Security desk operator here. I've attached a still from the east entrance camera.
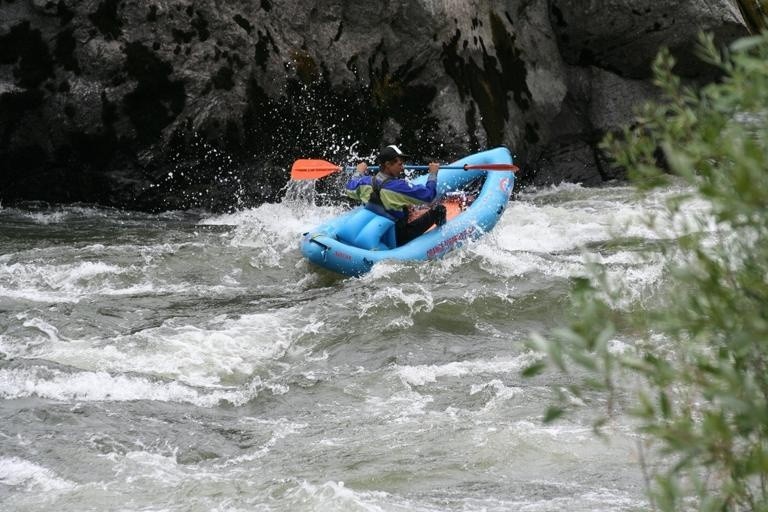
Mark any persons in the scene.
[343,145,446,247]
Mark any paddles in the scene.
[290,159,519,180]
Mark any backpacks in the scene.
[369,176,393,208]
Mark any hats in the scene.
[381,145,410,161]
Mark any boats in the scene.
[301,147,516,276]
